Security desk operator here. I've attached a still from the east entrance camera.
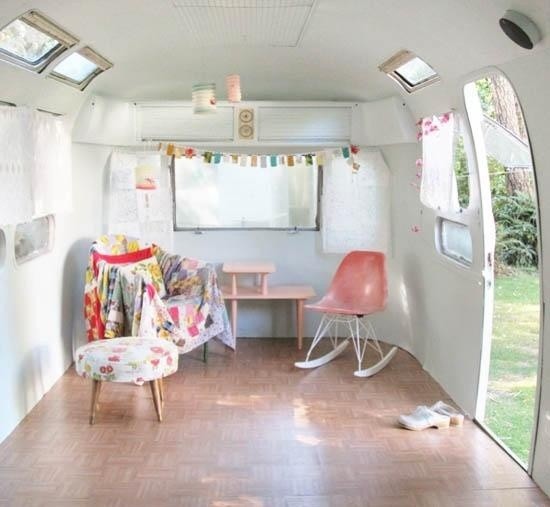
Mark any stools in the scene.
[76,336,178,424]
[222,260,276,295]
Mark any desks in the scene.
[219,285,316,350]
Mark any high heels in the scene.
[398,406,451,431]
[429,400,464,425]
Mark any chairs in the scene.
[294,251,398,378]
[84,233,218,362]
